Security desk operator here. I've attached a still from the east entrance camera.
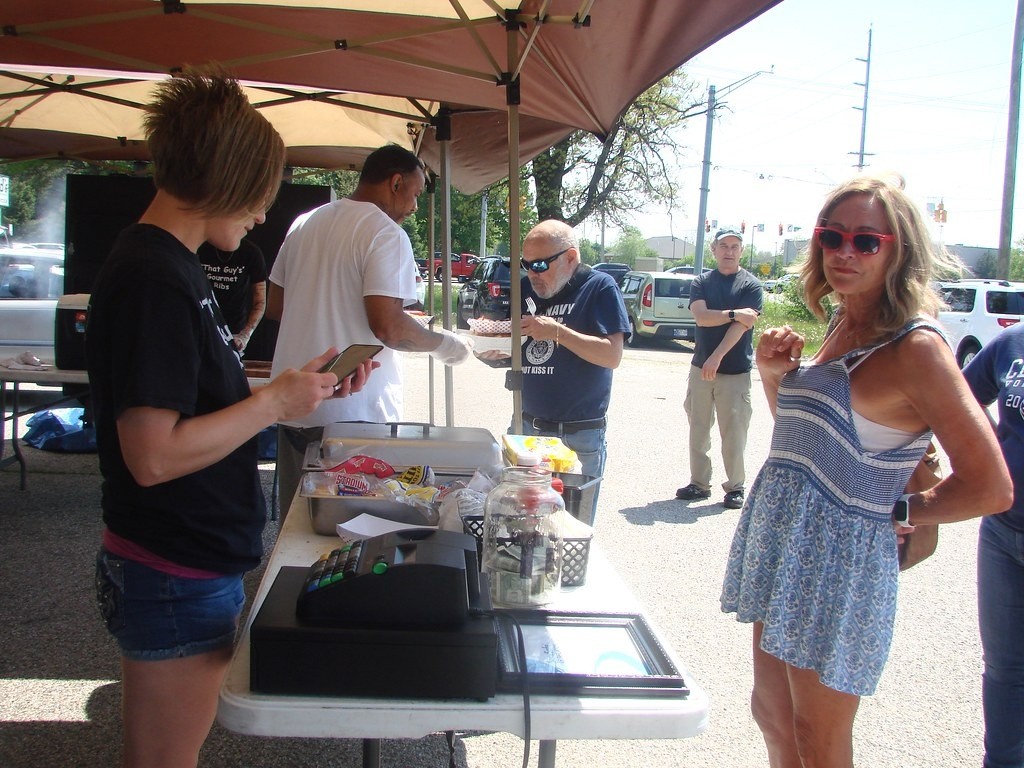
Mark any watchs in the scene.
[729,309,735,323]
[894,494,916,528]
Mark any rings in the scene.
[790,356,801,362]
[349,392,354,398]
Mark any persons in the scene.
[196,238,268,352]
[472,220,632,528]
[720,180,1014,768]
[83,61,380,768]
[961,322,1024,768]
[267,145,475,541]
[675,225,764,509]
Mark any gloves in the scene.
[428,329,474,367]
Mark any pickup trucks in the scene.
[426,253,481,283]
[415,251,447,278]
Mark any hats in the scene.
[715,226,743,241]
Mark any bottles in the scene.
[481,465,562,607]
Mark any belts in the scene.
[522,412,608,434]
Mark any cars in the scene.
[928,277,1024,372]
[1,242,89,390]
[664,266,713,276]
[619,271,701,346]
[592,263,632,285]
[765,274,799,293]
[455,257,531,329]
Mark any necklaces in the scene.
[844,317,876,340]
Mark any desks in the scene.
[0,363,278,521]
[217,473,705,768]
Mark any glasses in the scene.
[520,247,575,273]
[815,227,896,256]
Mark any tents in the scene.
[0,0,779,436]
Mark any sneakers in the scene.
[675,483,712,500]
[724,491,744,508]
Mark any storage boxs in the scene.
[55,293,90,370]
[461,515,593,586]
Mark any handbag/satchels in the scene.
[899,440,944,573]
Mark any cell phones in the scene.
[318,344,384,387]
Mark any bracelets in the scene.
[557,322,559,348]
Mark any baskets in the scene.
[461,515,595,586]
